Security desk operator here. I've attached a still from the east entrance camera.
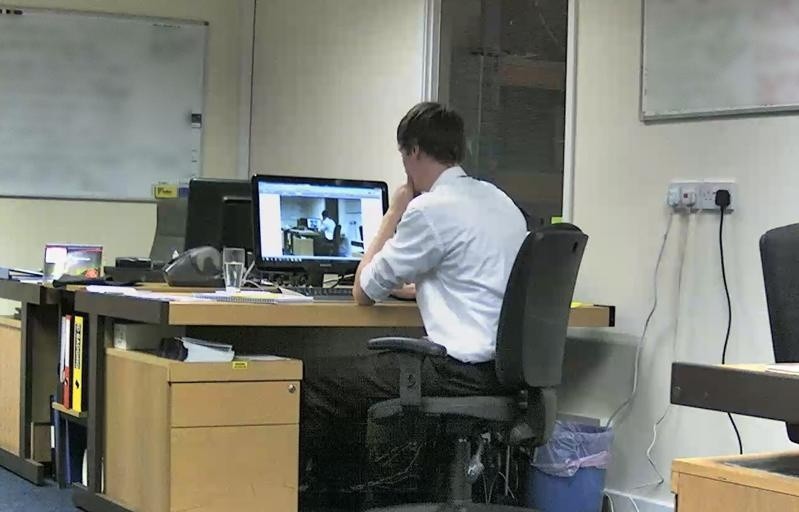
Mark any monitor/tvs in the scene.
[182,176,254,252]
[306,217,322,231]
[297,218,306,226]
[250,173,390,288]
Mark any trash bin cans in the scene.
[529,422,614,512]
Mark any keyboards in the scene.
[286,285,354,301]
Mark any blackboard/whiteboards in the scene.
[0,5,210,204]
[638,1,799,122]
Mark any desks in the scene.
[66,285,616,512]
[1,267,162,491]
[661,356,797,434]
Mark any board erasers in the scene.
[152,184,179,198]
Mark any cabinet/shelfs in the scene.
[670,451,798,511]
[2,316,54,462]
[103,345,304,512]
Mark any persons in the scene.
[302,102,527,509]
[317,210,337,250]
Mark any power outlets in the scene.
[701,180,735,211]
[667,182,699,211]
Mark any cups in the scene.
[222,246,255,294]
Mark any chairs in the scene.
[752,221,797,451]
[144,196,189,273]
[362,223,589,510]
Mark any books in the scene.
[58,317,65,404]
[71,314,86,411]
[6,267,43,277]
[63,314,74,410]
[193,292,313,305]
[159,335,236,362]
